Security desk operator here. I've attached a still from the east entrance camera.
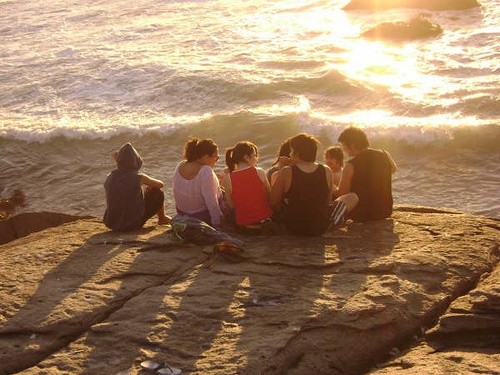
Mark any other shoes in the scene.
[158,216,171,224]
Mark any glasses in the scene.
[214,154,219,161]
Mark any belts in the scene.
[246,224,262,229]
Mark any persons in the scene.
[173,137,236,231]
[265,140,291,184]
[103,141,174,233]
[323,145,345,192]
[225,140,281,234]
[330,125,397,222]
[271,133,334,237]
